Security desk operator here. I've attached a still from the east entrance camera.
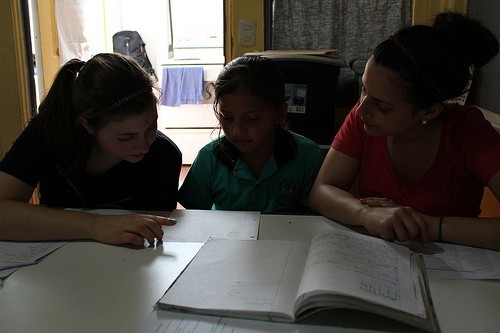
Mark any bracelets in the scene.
[438,216,444,243]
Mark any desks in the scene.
[0,210,500,333]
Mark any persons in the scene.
[309,9,500,253]
[0,54,182,247]
[178,57,324,215]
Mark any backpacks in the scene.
[113,30,152,74]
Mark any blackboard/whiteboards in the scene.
[169,0,224,49]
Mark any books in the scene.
[154,228,441,333]
